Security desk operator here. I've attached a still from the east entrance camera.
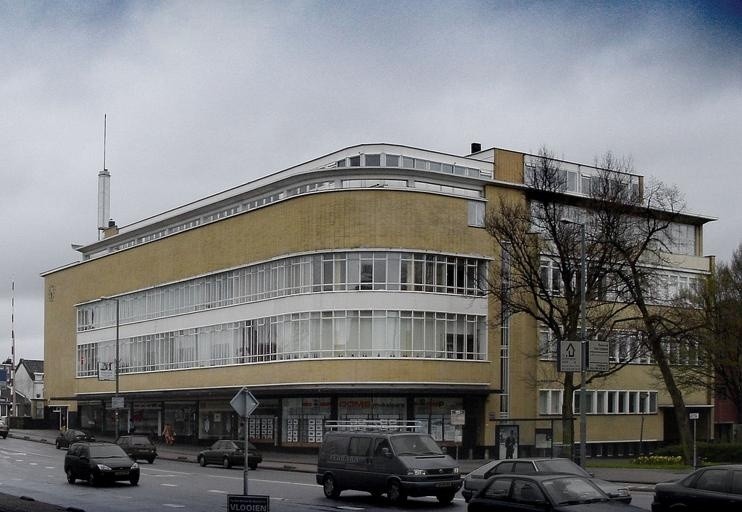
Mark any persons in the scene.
[238,423,244,440]
[60,424,69,437]
[161,423,172,445]
[504,429,516,459]
[130,417,135,435]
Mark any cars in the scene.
[462,457,650,511]
[651,465,742,512]
[115,435,158,464]
[196,439,263,470]
[0,418,8,439]
[55,428,96,450]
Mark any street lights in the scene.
[99,296,121,443]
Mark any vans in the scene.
[316,431,464,505]
[64,439,139,487]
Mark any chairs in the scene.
[520,481,567,500]
